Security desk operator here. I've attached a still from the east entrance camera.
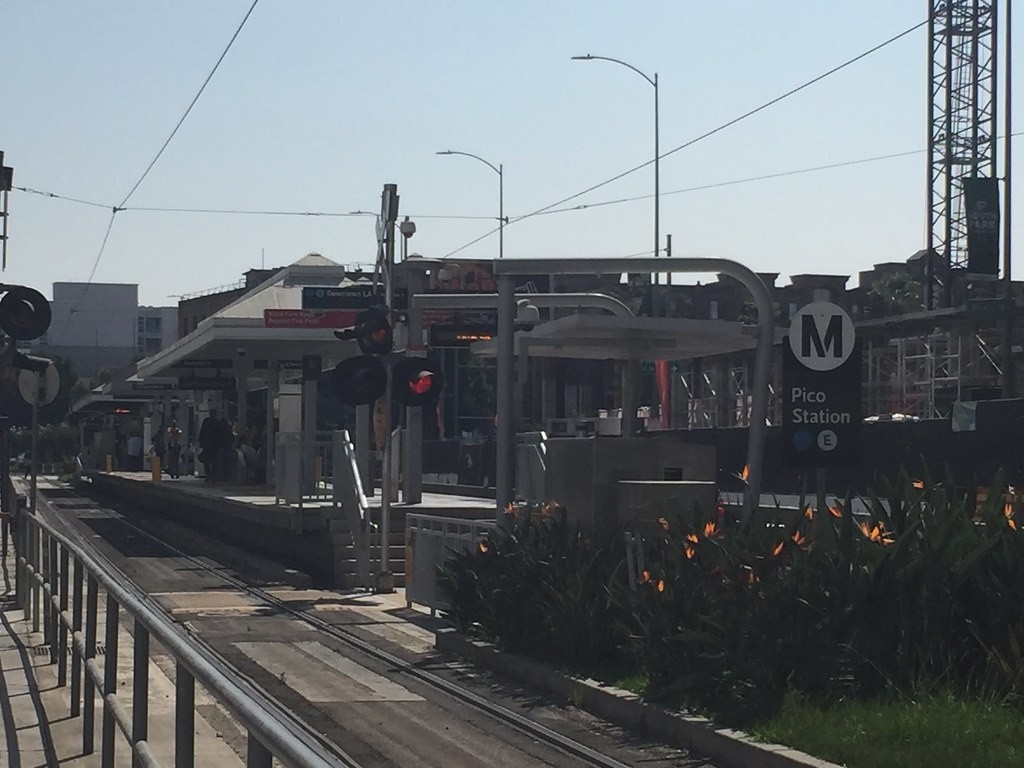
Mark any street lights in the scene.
[571,51,660,285]
[436,153,511,261]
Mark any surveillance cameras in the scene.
[517,306,540,332]
[400,222,416,238]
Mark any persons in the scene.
[127,418,183,479]
[198,407,234,485]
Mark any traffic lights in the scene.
[388,354,444,411]
[334,301,393,358]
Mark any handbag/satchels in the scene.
[198,447,208,462]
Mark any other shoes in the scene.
[204,474,211,483]
